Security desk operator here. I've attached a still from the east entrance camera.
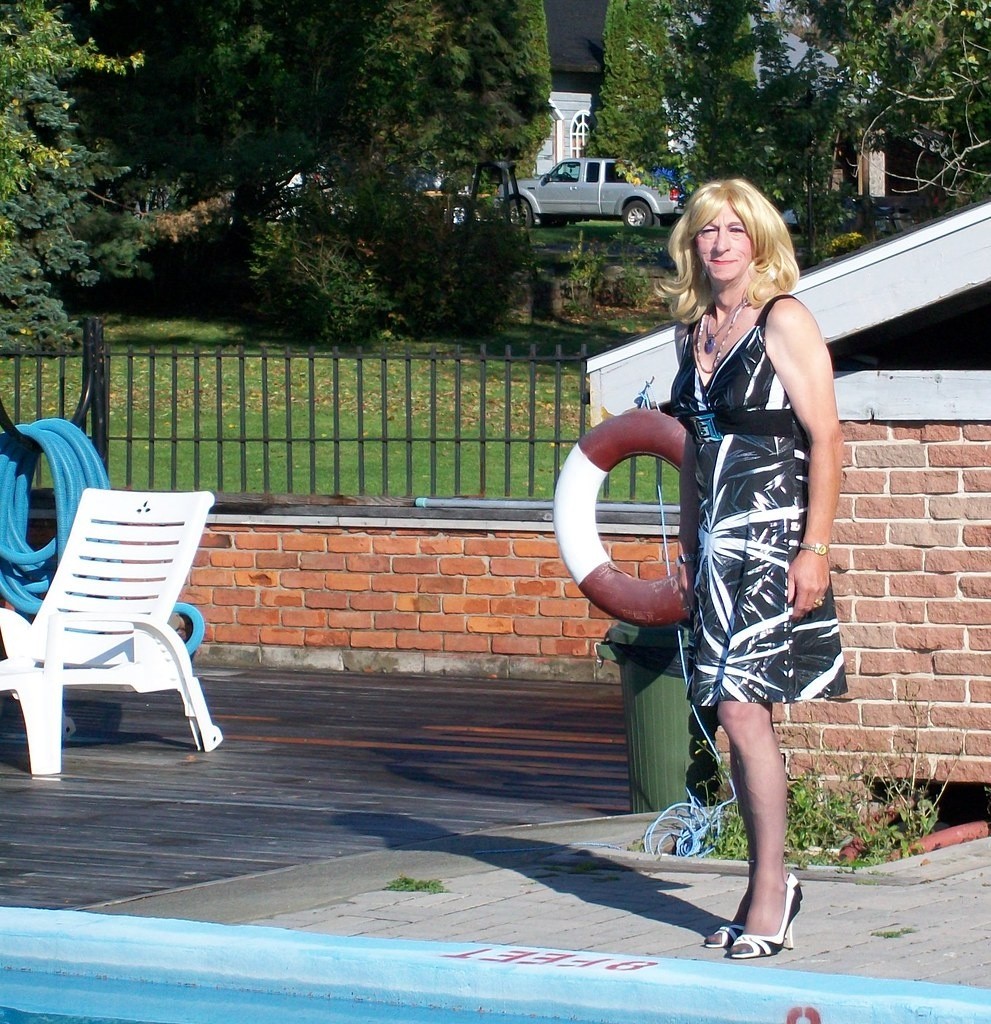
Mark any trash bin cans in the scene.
[596,621,719,814]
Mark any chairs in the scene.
[0,484,226,779]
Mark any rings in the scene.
[814,599,823,606]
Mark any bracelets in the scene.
[675,553,697,568]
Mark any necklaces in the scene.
[705,300,746,354]
[696,301,747,373]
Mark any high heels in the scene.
[731,882,800,959]
[705,873,803,949]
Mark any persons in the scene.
[654,180,849,958]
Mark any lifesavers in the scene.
[552,411,693,626]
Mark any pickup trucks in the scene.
[498,158,691,230]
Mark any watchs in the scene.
[800,542,828,555]
